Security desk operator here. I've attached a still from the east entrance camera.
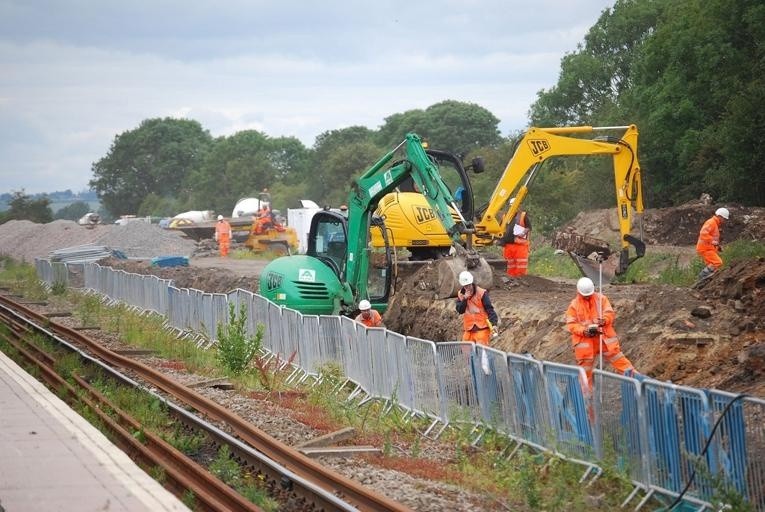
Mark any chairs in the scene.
[327,241,346,260]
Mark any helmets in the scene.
[358,299,372,311]
[714,207,730,220]
[458,271,474,286]
[217,215,224,221]
[576,277,594,297]
[509,198,516,205]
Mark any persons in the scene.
[694,207,730,278]
[493,197,533,276]
[353,299,382,336]
[251,204,287,235]
[455,271,499,371]
[215,215,231,257]
[566,277,640,426]
